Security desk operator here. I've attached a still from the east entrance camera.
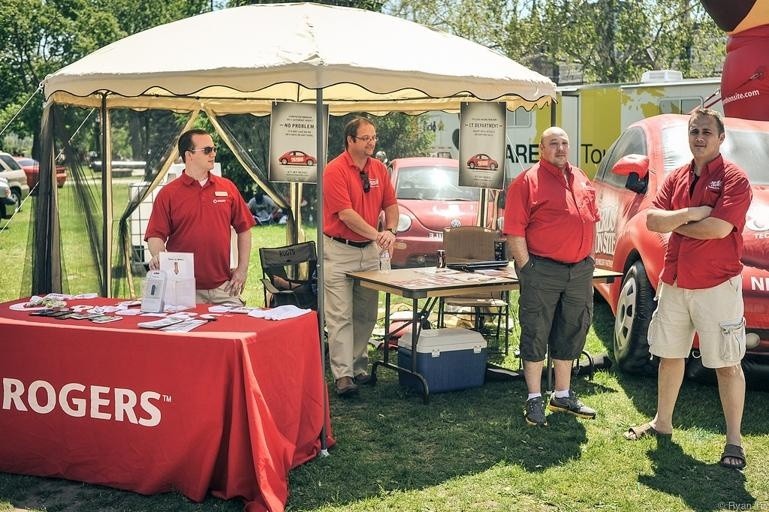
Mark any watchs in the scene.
[382,226,396,235]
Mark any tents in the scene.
[39,0,559,393]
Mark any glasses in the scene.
[360,171,370,193]
[188,147,217,155]
[350,135,379,142]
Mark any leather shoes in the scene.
[353,373,378,385]
[335,376,360,396]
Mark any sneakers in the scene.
[548,389,596,418]
[523,396,548,426]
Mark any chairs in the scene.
[438,226,510,355]
[259,242,330,348]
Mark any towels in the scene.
[248,304,312,321]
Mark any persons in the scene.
[145,129,258,310]
[316,119,401,396]
[619,107,754,469]
[503,126,601,427]
[246,186,276,226]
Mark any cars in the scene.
[378,157,504,268]
[467,152,499,171]
[593,111,768,378]
[13,156,67,192]
[279,150,318,167]
[0,152,30,214]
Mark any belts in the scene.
[529,253,588,265]
[323,232,373,248]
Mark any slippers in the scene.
[622,423,672,440]
[720,444,746,468]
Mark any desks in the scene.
[0,292,318,499]
[342,266,624,405]
